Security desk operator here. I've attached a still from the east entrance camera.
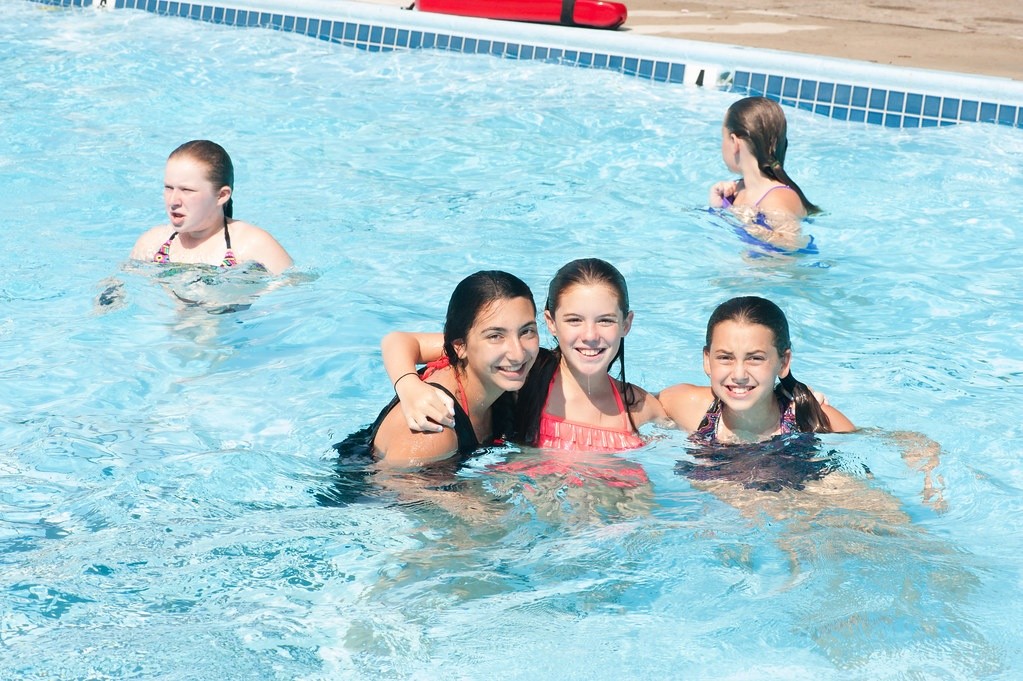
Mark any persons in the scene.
[709,96,822,216]
[381,258,672,459]
[333,270,539,469]
[129,140,292,276]
[653,296,856,443]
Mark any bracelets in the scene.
[393,371,423,390]
